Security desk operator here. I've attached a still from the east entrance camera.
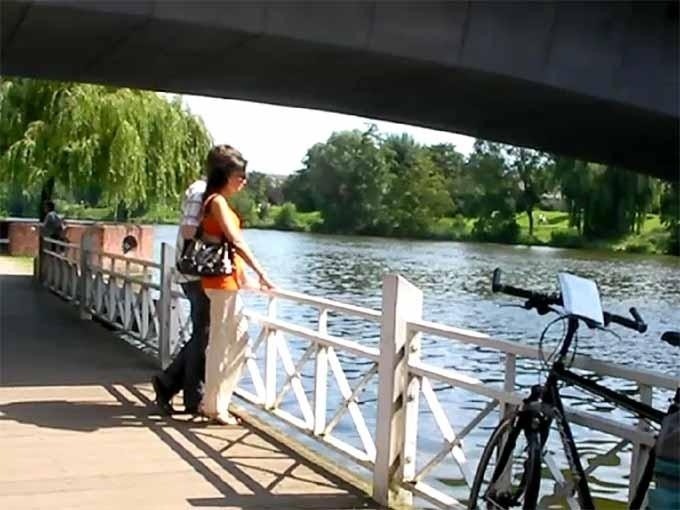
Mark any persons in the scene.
[197,147,276,427]
[151,143,242,418]
[32,200,66,258]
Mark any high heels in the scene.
[197,402,242,424]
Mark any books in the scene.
[557,269,607,327]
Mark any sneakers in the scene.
[185,403,200,414]
[152,376,176,415]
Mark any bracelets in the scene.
[258,271,265,279]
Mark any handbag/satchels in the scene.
[176,238,234,276]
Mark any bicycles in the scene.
[466,266,679,509]
